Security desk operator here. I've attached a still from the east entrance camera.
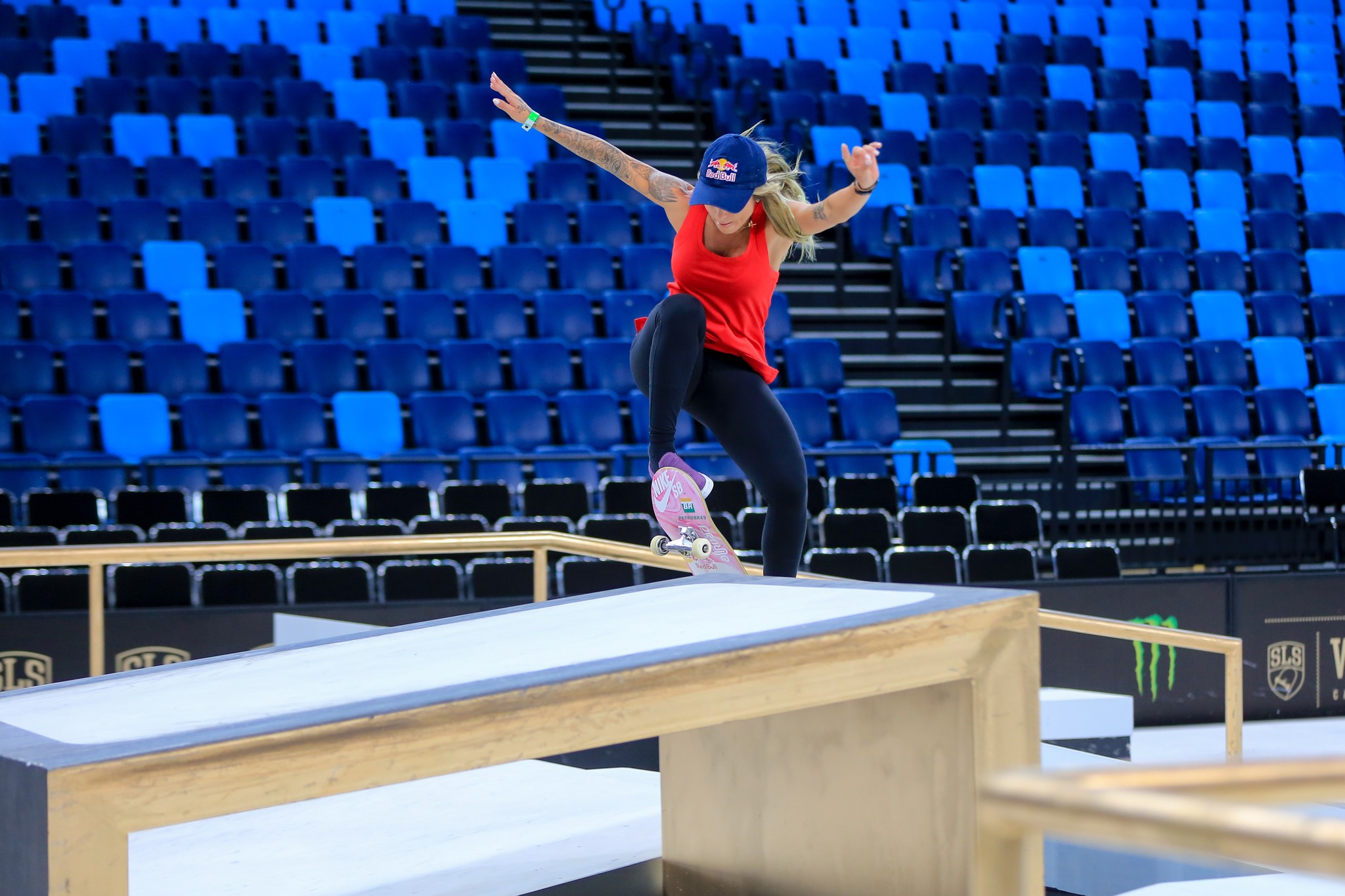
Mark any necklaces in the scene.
[737,220,757,230]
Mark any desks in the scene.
[0,571,1047,895]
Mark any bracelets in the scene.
[853,181,878,195]
[521,110,540,132]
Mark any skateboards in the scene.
[650,466,748,576]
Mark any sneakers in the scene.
[648,452,713,499]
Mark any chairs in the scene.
[0,1,1345,618]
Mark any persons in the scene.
[490,71,882,577]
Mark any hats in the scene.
[689,134,767,214]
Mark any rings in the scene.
[844,156,850,162]
